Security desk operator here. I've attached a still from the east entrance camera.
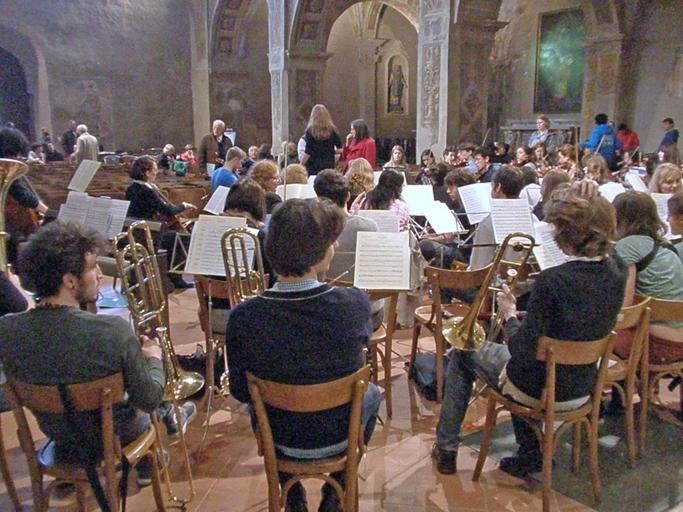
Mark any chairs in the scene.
[244,363,372,512]
[408,262,496,403]
[19,148,212,218]
[571,295,654,470]
[321,276,402,417]
[500,259,540,280]
[0,372,165,511]
[471,330,618,512]
[193,273,270,353]
[633,290,683,458]
[0,433,23,511]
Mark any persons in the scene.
[282,163,307,185]
[279,144,299,168]
[457,143,479,175]
[473,146,497,182]
[582,151,611,186]
[313,170,385,333]
[248,160,282,215]
[641,153,659,187]
[417,150,439,185]
[528,116,558,155]
[515,146,536,170]
[0,223,196,485]
[180,145,202,173]
[581,114,620,173]
[1,128,48,263]
[257,144,273,160]
[342,158,374,211]
[383,145,416,184]
[445,168,481,241]
[298,104,342,175]
[649,163,683,194]
[614,124,640,160]
[657,118,678,154]
[349,170,410,233]
[609,191,682,399]
[62,120,80,160]
[198,179,275,347]
[494,142,512,163]
[533,143,546,168]
[226,198,380,511]
[243,145,257,167]
[44,144,62,162]
[211,147,246,196]
[71,124,99,163]
[198,120,233,172]
[657,146,677,164]
[533,172,572,221]
[622,149,638,179]
[27,143,46,164]
[431,184,628,475]
[157,144,180,174]
[123,155,197,288]
[517,167,539,198]
[557,145,583,182]
[438,146,459,173]
[342,118,376,175]
[420,168,525,306]
[664,192,683,255]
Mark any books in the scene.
[490,199,537,244]
[355,231,412,291]
[56,190,131,242]
[650,192,683,240]
[424,199,465,235]
[625,174,648,195]
[599,182,626,202]
[184,214,259,278]
[67,160,102,193]
[457,182,498,224]
[400,183,435,216]
[533,223,577,271]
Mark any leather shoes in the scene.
[500,449,558,477]
[432,442,457,474]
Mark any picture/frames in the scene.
[532,7,585,114]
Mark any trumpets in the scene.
[221,228,266,309]
[0,158,29,281]
[115,220,206,507]
[441,233,534,351]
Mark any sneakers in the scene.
[163,400,199,439]
[135,447,170,487]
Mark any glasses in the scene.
[270,175,283,184]
